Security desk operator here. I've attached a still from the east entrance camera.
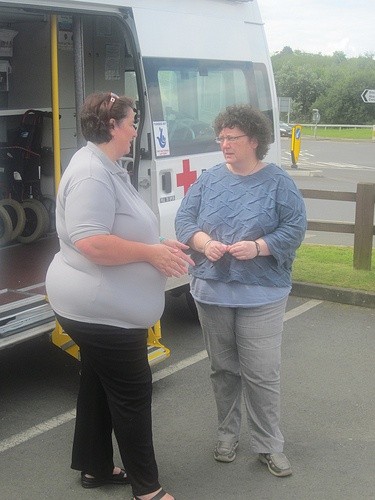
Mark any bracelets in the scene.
[203,240,212,254]
[255,242,260,256]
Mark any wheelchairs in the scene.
[0,109,60,245]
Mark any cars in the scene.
[279,121,293,137]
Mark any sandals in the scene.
[133,489,175,500]
[80,466,129,488]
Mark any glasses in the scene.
[216,134,246,143]
[107,91,120,110]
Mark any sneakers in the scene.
[259,453,292,477]
[214,439,240,461]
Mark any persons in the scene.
[175,104,308,476]
[45,92,194,500]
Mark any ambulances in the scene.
[0,0,281,352]
